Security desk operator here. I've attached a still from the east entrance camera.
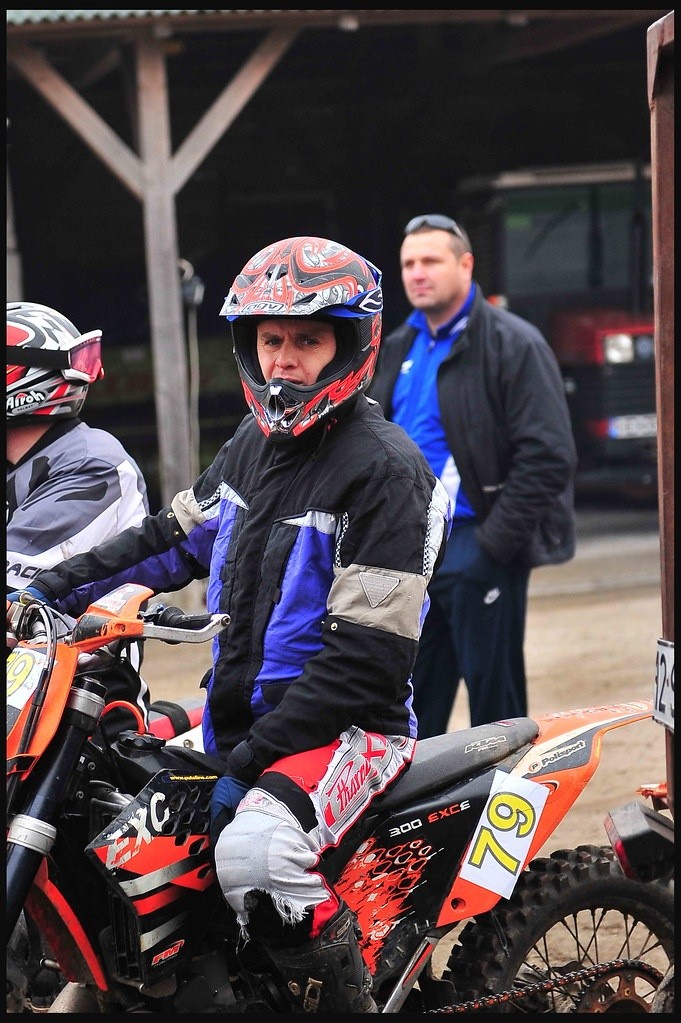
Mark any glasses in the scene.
[404,213,470,251]
[325,255,382,319]
[6,330,104,384]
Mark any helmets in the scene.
[6,302,90,428]
[218,236,383,444]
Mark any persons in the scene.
[365,213,579,740]
[6,234,452,1013]
[6,300,152,733]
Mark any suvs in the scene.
[459,159,658,485]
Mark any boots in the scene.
[264,907,379,1014]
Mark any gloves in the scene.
[209,739,262,851]
[6,579,56,628]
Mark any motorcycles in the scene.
[4,580,677,1011]
